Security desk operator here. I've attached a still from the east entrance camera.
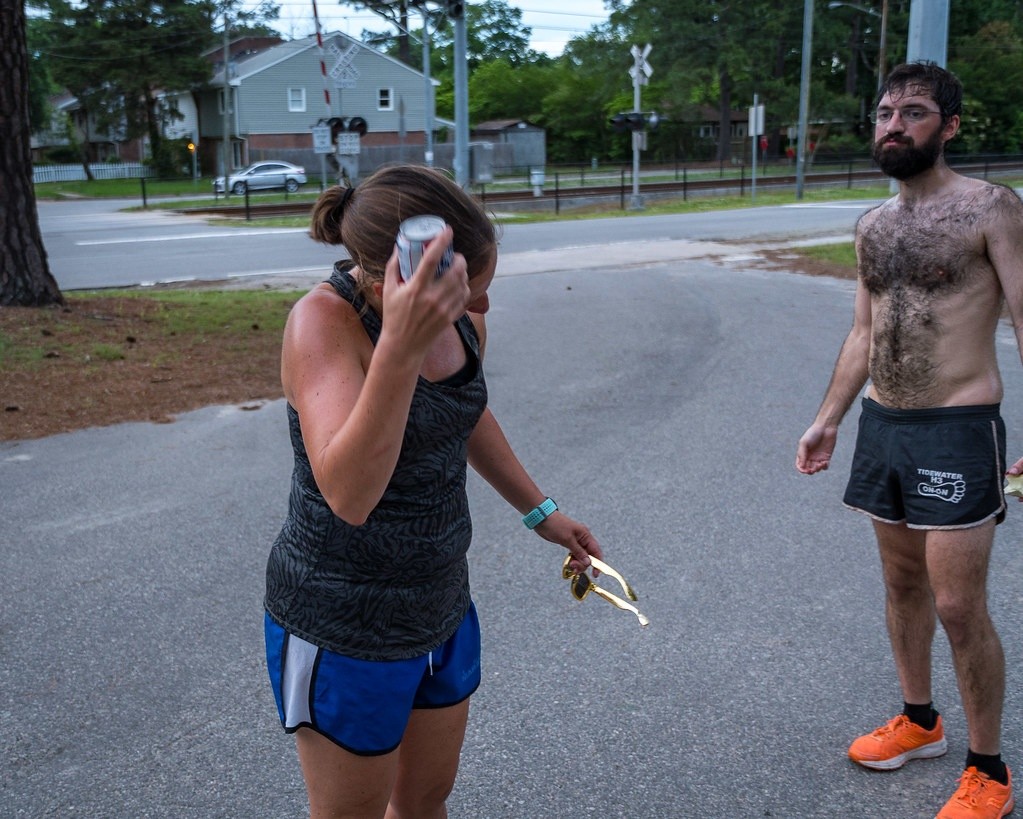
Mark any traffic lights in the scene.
[608,113,644,131]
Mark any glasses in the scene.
[562,551,649,625]
[868,107,942,124]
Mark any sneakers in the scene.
[848,714,948,769]
[934,762,1015,819]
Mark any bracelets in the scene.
[520,496,559,531]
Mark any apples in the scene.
[1003,472,1023,498]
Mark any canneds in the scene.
[395,215,452,283]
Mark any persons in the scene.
[795,57,1023,819]
[263,166,605,819]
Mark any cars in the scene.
[211,158,308,197]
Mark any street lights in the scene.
[827,2,888,170]
